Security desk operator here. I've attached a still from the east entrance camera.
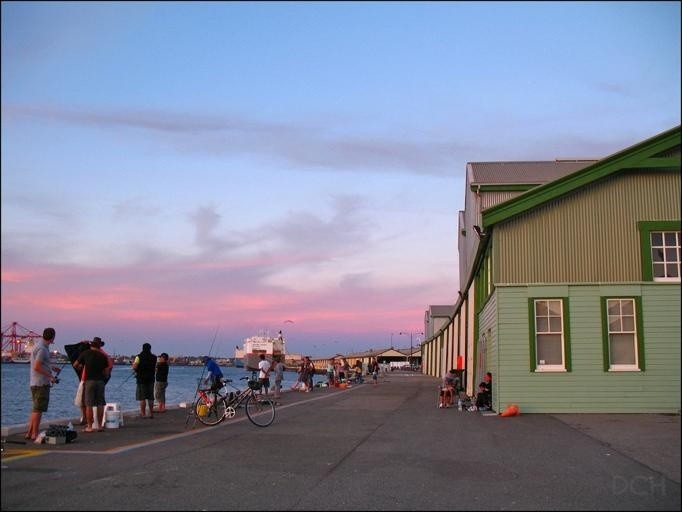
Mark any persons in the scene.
[76,340,112,433]
[132,342,156,419]
[326,357,379,385]
[258,354,271,396]
[24,328,62,439]
[479,381,486,392]
[273,360,284,398]
[200,355,223,393]
[292,356,315,392]
[438,370,461,409]
[154,352,169,412]
[467,373,492,412]
[72,336,114,425]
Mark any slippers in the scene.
[24,407,166,440]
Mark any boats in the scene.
[235,336,285,371]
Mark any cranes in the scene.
[1,322,42,352]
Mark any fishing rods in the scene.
[185,328,216,425]
[112,371,136,393]
[54,357,70,378]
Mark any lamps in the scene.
[472,224,487,238]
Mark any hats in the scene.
[158,352,168,358]
[201,356,209,363]
[89,337,105,347]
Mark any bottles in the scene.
[252,372,257,381]
[66,421,74,431]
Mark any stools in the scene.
[101,403,124,429]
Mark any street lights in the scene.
[400,332,423,371]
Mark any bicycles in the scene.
[195,367,275,427]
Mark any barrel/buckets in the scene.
[103,410,122,430]
[196,404,210,417]
